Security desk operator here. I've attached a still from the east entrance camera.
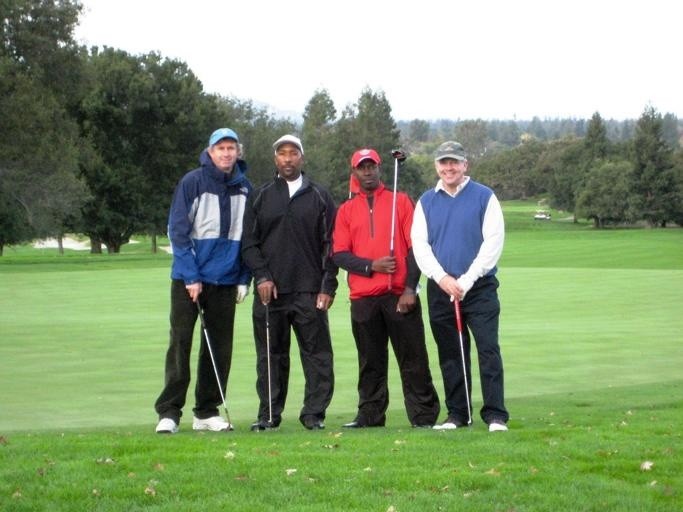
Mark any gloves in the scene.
[450,274,474,303]
[236,284,248,304]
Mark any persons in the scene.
[332,148,440,429]
[154,125,255,434]
[412,139,511,433]
[241,134,338,431]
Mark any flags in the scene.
[349,173,361,194]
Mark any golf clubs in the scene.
[195,299,232,433]
[453,299,473,427]
[387,150,407,293]
[259,305,274,429]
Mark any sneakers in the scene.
[305,422,325,428]
[251,421,279,431]
[155,418,178,433]
[343,421,360,427]
[433,416,465,430]
[489,419,508,432]
[192,415,233,431]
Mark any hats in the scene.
[272,135,304,156]
[209,127,238,144]
[350,148,382,168]
[434,141,465,162]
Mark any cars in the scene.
[534,210,552,220]
